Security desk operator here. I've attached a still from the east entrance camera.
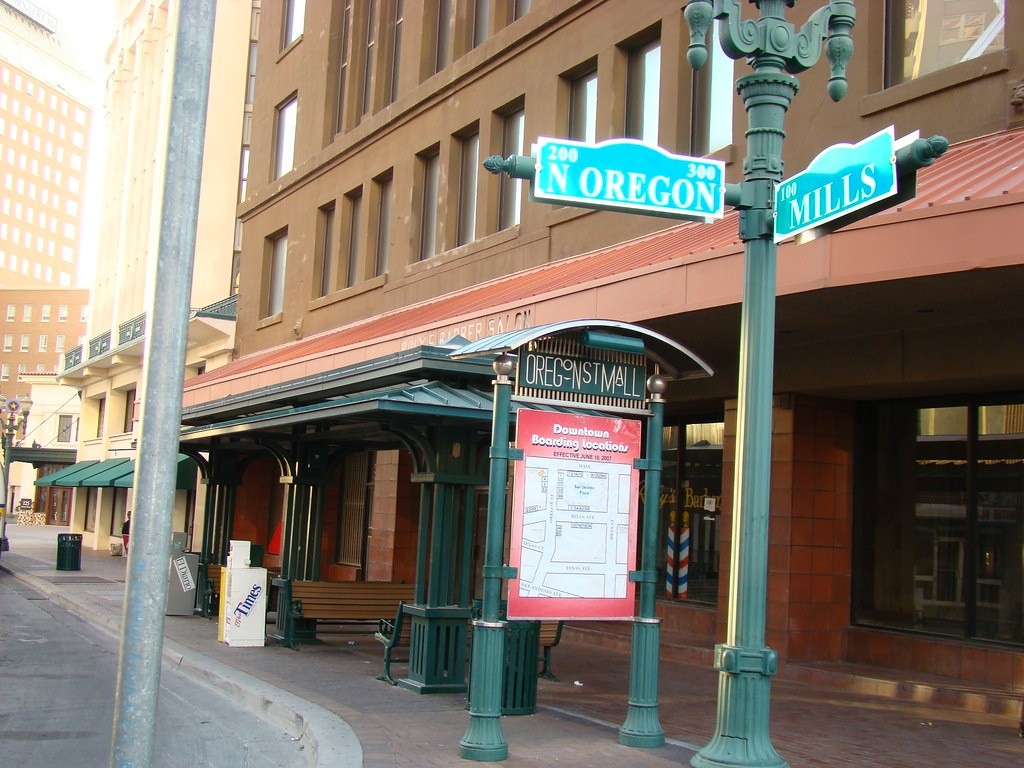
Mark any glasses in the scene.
[127,514,130,515]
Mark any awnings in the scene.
[34,456,189,488]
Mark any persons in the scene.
[121,510,131,555]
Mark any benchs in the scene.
[282,581,416,651]
[205,564,277,617]
[375,600,564,685]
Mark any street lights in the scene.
[0,393,33,552]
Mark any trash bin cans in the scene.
[56,533,82,570]
[465,597,542,716]
